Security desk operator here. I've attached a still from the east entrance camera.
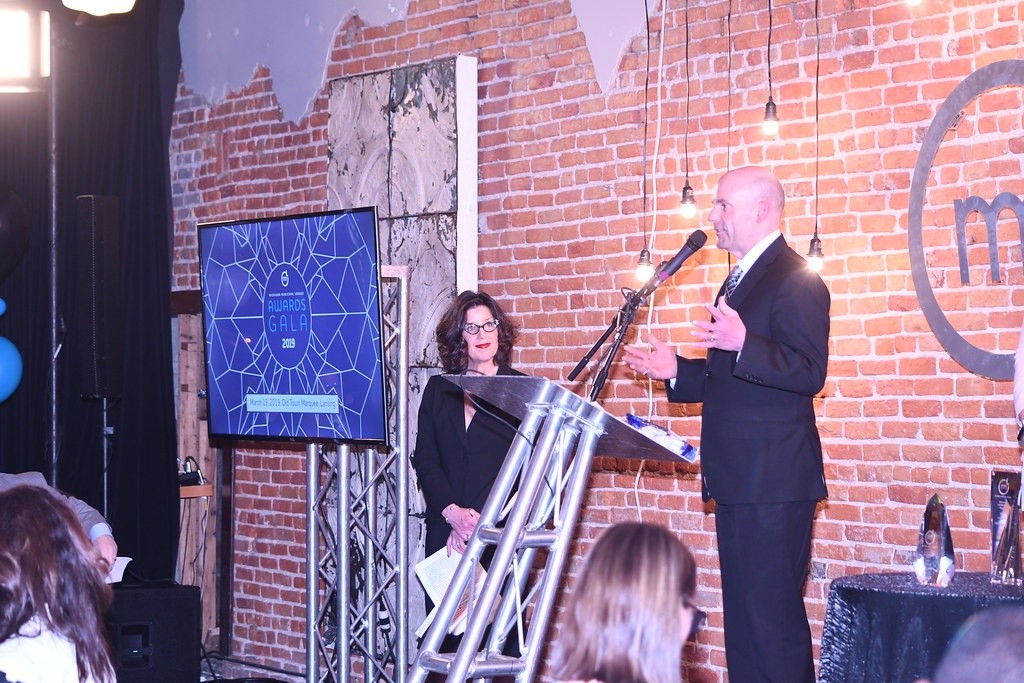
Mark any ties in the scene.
[724,266,742,301]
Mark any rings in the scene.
[456,543,460,547]
[643,371,648,376]
[467,535,471,539]
[710,333,715,341]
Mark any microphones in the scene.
[644,230,708,299]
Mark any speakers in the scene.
[101,583,201,683]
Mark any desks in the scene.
[818,569,1024,683]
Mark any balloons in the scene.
[0,299,6,315]
[0,336,22,402]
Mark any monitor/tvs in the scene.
[197,206,390,446]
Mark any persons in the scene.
[552,521,697,683]
[622,166,831,683]
[0,472,117,568]
[415,290,531,683]
[915,606,1024,683]
[0,484,117,683]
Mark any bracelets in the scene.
[446,506,459,523]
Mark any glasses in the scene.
[682,600,707,633]
[464,319,500,334]
